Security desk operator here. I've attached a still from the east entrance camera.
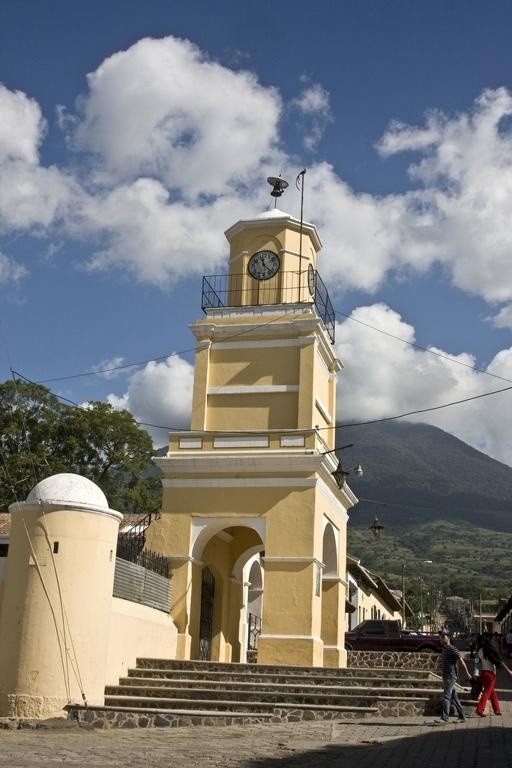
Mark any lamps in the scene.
[358,498,388,538]
[320,442,364,491]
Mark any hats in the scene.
[439,628,454,638]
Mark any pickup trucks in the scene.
[344,618,454,652]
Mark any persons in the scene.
[435,629,473,724]
[473,633,511,717]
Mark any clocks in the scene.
[247,250,280,280]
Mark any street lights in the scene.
[398,560,432,627]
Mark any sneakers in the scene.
[478,712,486,717]
[496,713,502,715]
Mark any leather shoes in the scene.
[434,718,448,723]
[453,719,465,723]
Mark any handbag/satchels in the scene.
[474,657,481,677]
